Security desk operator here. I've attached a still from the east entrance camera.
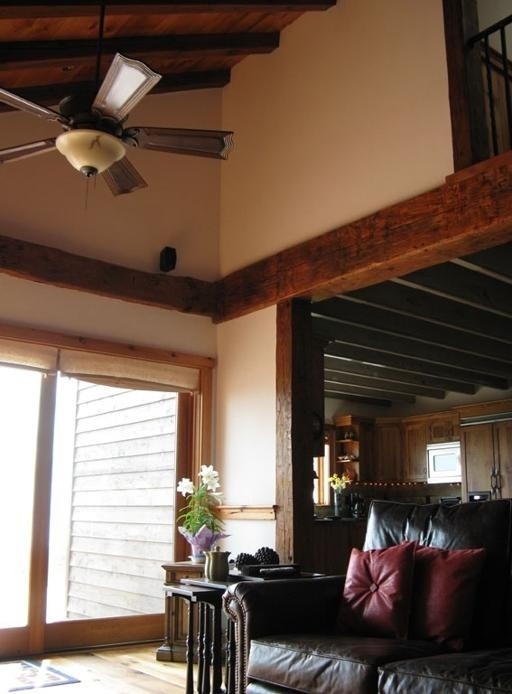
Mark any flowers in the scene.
[328,474,351,494]
[175,464,227,534]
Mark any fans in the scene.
[1,0,236,198]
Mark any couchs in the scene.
[220,500,512,694]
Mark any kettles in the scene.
[351,496,368,518]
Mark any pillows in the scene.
[339,541,417,641]
[411,544,486,650]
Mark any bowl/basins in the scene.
[188,554,206,563]
[338,453,358,462]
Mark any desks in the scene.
[164,567,261,694]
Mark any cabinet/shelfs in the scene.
[403,409,461,483]
[452,398,512,503]
[333,413,376,482]
[376,415,403,482]
[156,561,236,662]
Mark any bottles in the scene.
[348,430,354,440]
[343,430,350,440]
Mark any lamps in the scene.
[53,128,126,180]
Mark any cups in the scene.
[202,544,233,581]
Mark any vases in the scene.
[334,493,342,517]
[177,525,230,563]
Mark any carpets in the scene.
[0,655,83,693]
